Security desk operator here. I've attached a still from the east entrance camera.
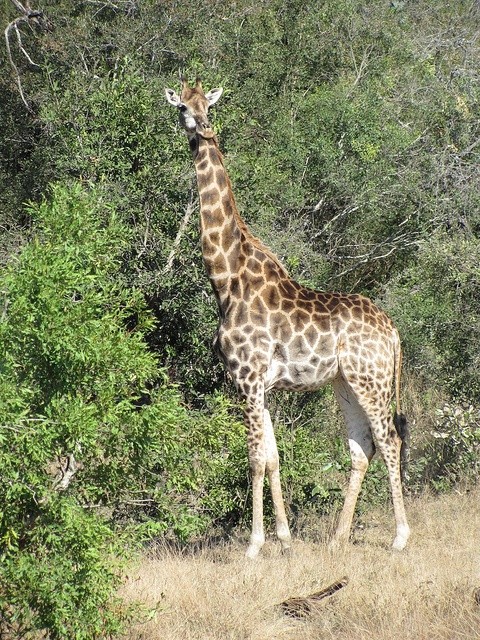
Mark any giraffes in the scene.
[162,74,413,560]
